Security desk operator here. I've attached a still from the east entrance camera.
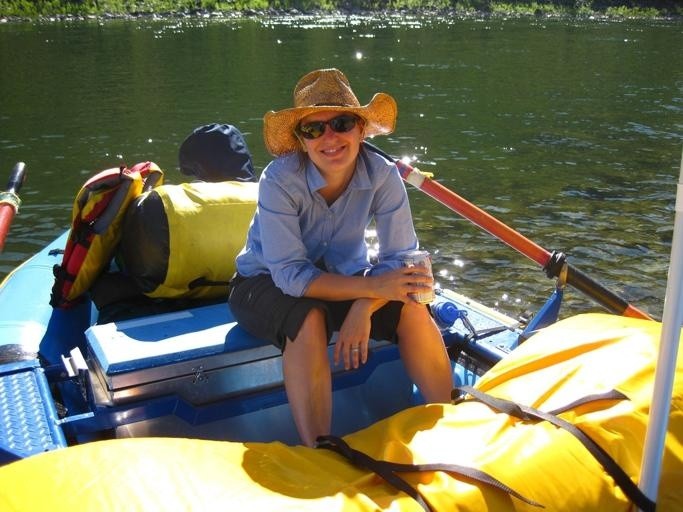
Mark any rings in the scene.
[352,348,359,353]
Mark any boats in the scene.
[0,211,572,470]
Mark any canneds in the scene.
[401,251,435,305]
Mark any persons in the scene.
[228,68,455,448]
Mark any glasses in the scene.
[300,115,360,138]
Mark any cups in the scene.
[431,302,468,328]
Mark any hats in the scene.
[264,68,396,156]
